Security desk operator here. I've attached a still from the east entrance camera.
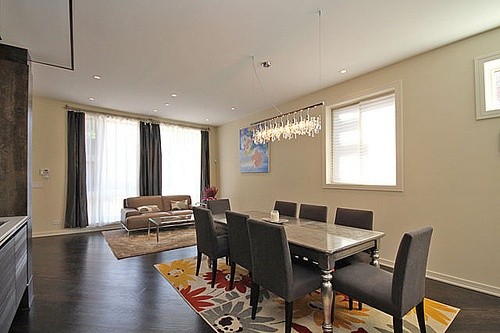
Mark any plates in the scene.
[262,218,290,223]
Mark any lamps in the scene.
[250,57,324,145]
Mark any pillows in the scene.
[138,205,160,214]
[170,199,189,210]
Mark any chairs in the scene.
[207,199,230,215]
[335,208,433,333]
[299,204,327,223]
[247,218,334,333]
[192,206,230,288]
[225,210,250,289]
[273,201,297,217]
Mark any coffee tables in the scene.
[149,214,195,242]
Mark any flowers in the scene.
[200,186,218,203]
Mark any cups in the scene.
[271,210,279,221]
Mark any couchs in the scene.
[121,195,194,236]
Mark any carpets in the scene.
[102,226,197,259]
[153,252,461,333]
